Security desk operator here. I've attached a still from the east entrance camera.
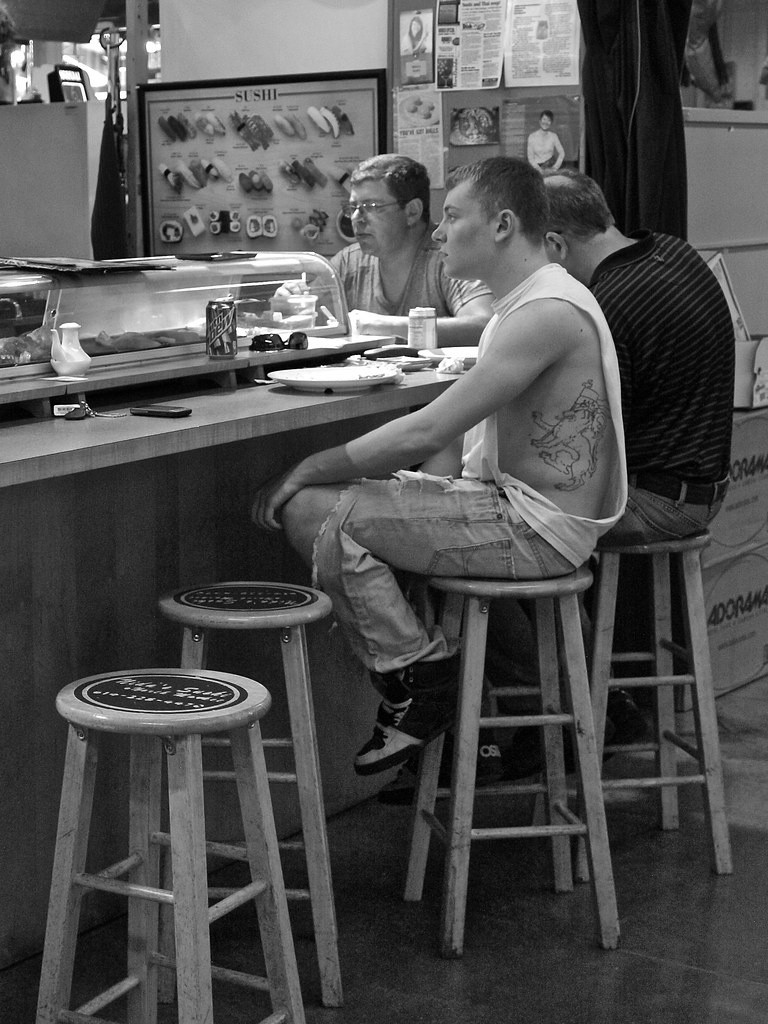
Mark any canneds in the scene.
[205,296,238,360]
[408,307,438,350]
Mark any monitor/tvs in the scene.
[46,63,93,104]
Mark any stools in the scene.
[531,527,734,885]
[157,579,344,1009]
[400,565,622,960]
[35,668,306,1024]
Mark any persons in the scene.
[496,166,735,782]
[527,109,565,177]
[249,157,628,805]
[275,153,494,347]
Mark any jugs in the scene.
[49,322,92,377]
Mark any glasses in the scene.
[249,332,308,351]
[341,199,415,218]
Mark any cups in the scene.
[288,295,318,328]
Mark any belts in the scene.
[629,472,730,505]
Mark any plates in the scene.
[267,366,397,395]
[347,358,430,372]
[417,347,478,369]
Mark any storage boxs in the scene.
[697,244,768,337]
[674,545,768,712]
[682,106,768,247]
[673,408,768,561]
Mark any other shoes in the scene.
[603,690,654,745]
[502,725,576,781]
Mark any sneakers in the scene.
[377,736,498,806]
[354,658,489,776]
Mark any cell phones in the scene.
[130,404,192,418]
